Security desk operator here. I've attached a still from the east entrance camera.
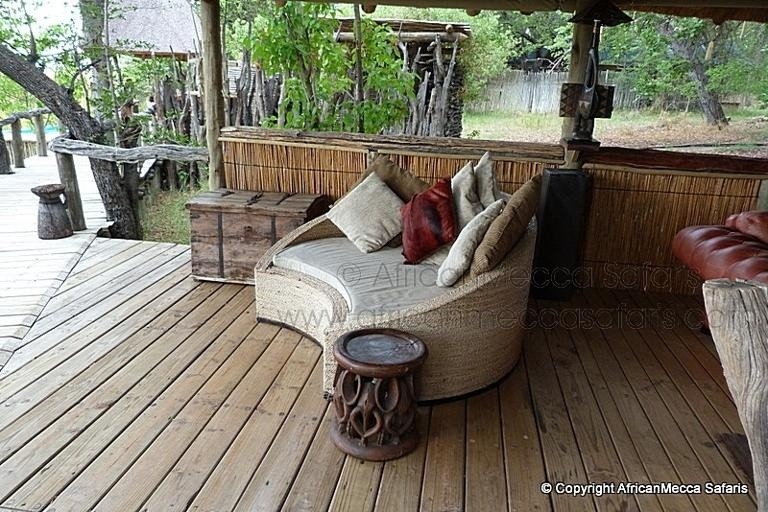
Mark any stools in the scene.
[329,326,429,462]
[30,183,73,240]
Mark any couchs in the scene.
[253,191,537,405]
[675,198,764,333]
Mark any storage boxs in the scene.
[185,187,324,287]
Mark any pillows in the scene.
[435,199,504,288]
[324,171,404,254]
[398,175,457,266]
[474,150,498,207]
[453,173,541,288]
[329,153,432,248]
[451,161,484,237]
